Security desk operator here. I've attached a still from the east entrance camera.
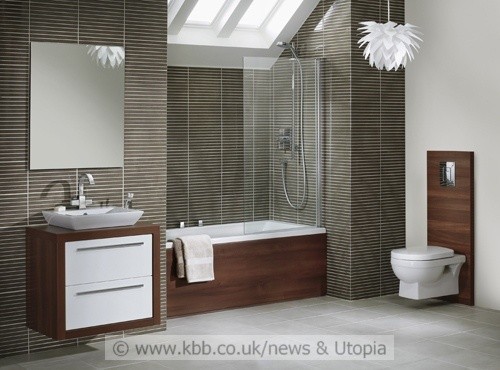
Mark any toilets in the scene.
[390,246,466,300]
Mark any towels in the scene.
[55,205,66,214]
[172,235,216,283]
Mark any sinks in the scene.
[42,206,144,231]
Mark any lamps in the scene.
[80,44,126,69]
[355,0,425,74]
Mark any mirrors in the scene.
[29,40,125,172]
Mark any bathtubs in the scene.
[166,220,327,317]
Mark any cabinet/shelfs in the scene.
[25,223,161,340]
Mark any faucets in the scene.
[70,172,95,210]
